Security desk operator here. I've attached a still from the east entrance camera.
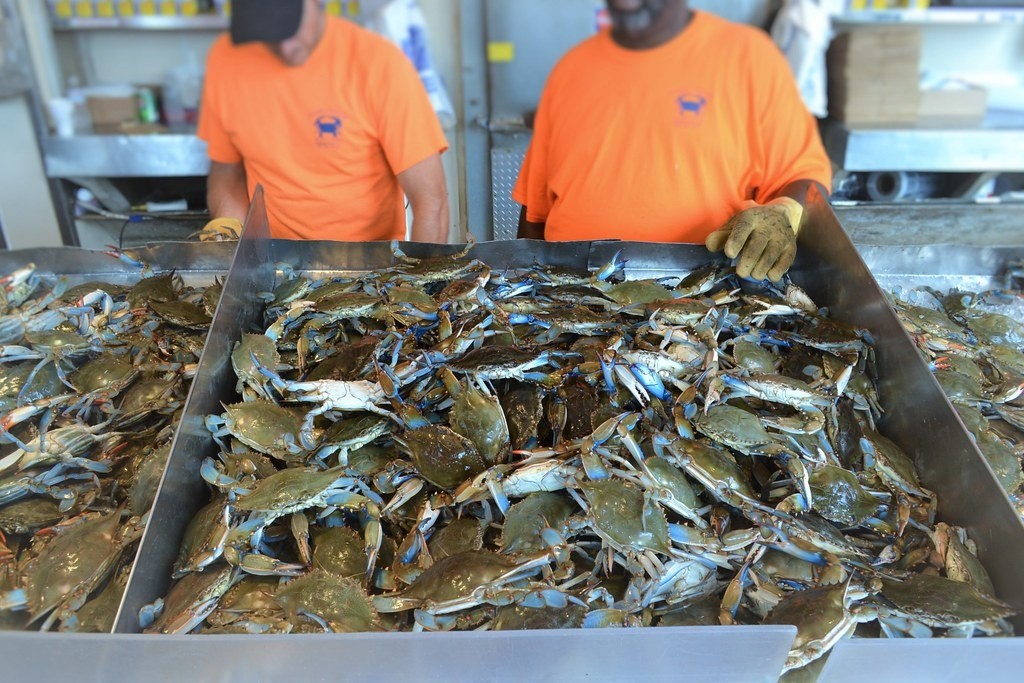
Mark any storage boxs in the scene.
[918,86,989,118]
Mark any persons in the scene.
[511,0,832,283]
[196,0,450,244]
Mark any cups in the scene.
[53,98,76,139]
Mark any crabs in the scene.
[0,238,1024,678]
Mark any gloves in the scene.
[704,197,804,283]
[199,217,242,242]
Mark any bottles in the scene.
[65,75,93,131]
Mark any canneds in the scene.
[135,87,161,123]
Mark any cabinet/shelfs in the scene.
[0,0,362,254]
[811,0,1024,206]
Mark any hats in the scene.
[232,0,302,44]
